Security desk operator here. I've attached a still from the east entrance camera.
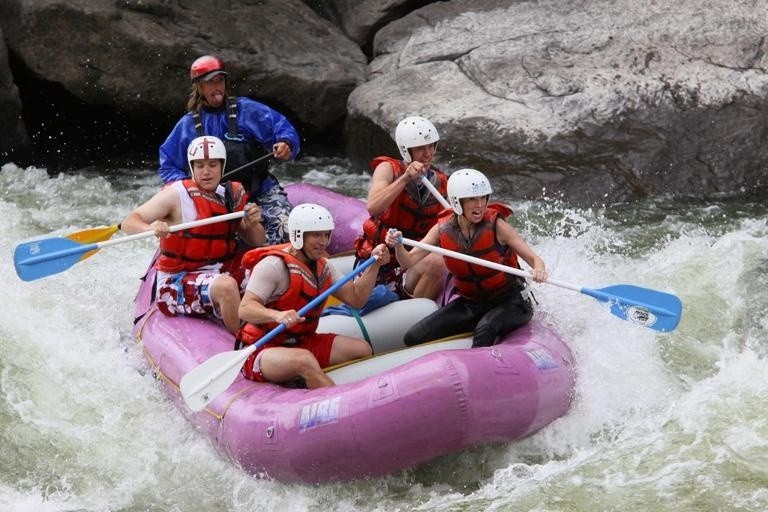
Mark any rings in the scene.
[287,318,292,321]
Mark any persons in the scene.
[352,116,450,287]
[382,167,548,350]
[233,202,392,391]
[121,135,265,336]
[157,55,302,247]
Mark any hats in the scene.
[194,70,229,82]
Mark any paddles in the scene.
[14,202,251,281]
[392,228,682,333]
[67,146,278,262]
[179,254,379,412]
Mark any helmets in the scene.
[446,169,492,216]
[395,116,440,163]
[288,203,335,250]
[190,55,225,84]
[186,135,227,180]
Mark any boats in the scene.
[130,179,582,487]
